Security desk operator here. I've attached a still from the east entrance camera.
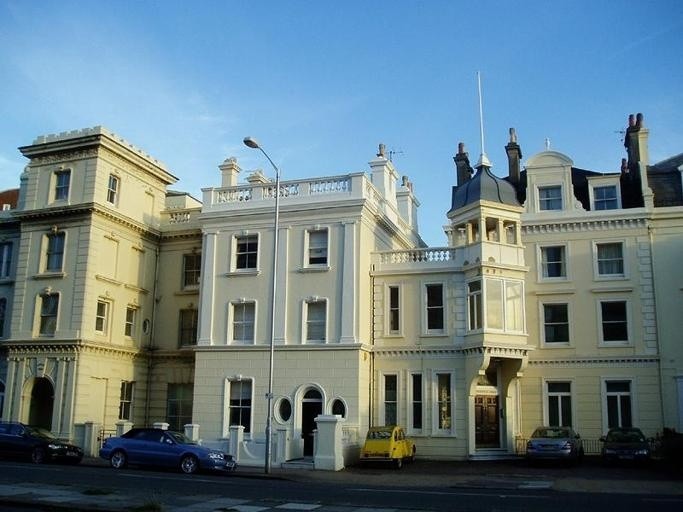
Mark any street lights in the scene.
[243,137,281,473]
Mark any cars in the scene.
[99,427,237,474]
[1,420,84,465]
[360,425,416,470]
[527,426,585,465]
[599,427,654,465]
